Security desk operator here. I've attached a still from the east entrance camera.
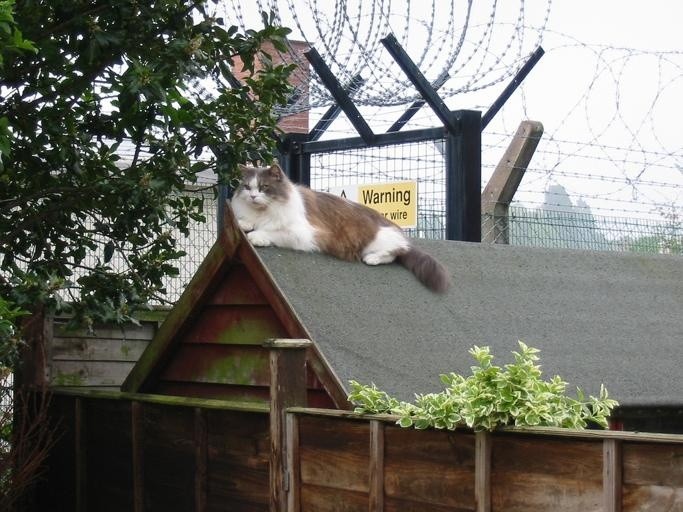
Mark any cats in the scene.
[230,162,453,298]
